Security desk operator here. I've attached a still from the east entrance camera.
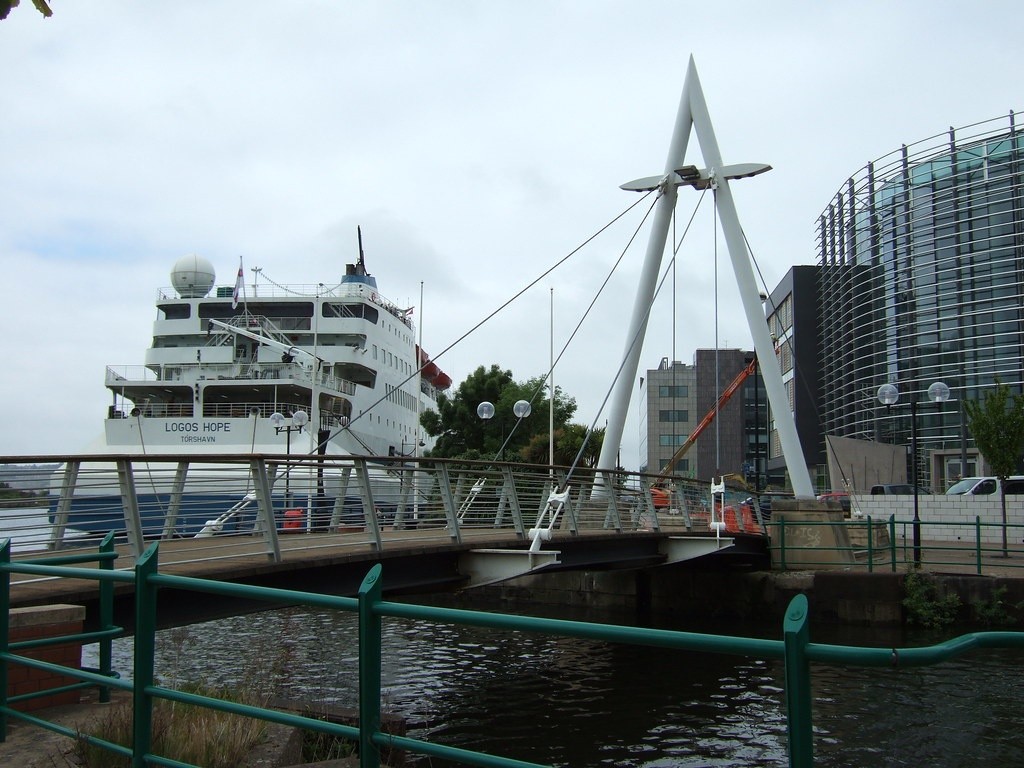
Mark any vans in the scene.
[945,475,1024,495]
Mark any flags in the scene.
[232,259,244,309]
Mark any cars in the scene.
[871,483,933,494]
[817,492,855,517]
[620,485,796,536]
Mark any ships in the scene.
[47,224,452,537]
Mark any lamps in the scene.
[673,165,700,180]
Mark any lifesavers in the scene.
[372,293,375,301]
[250,406,259,415]
[132,408,140,416]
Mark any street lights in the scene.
[270,411,308,507]
[400,438,426,493]
[753,291,767,501]
[877,382,950,568]
[477,400,531,461]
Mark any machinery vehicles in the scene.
[648,350,782,512]
